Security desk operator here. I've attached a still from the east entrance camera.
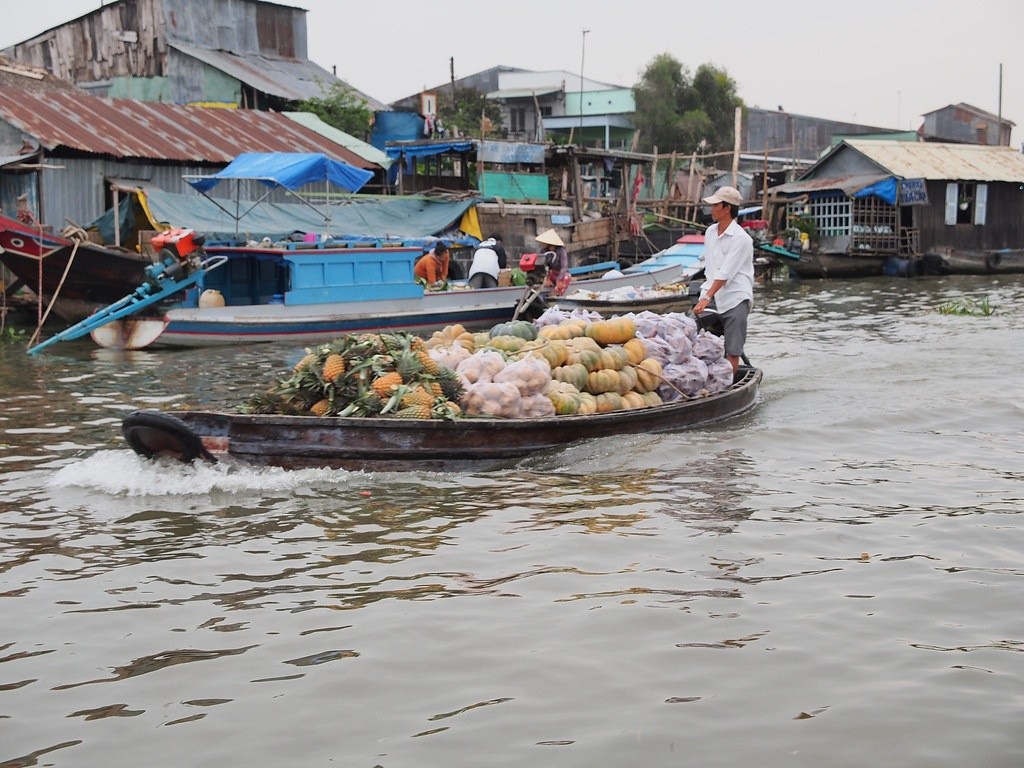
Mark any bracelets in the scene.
[702,295,712,303]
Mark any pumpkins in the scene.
[430,318,662,413]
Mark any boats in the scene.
[927,245,1024,270]
[121,354,774,469]
[546,279,700,316]
[89,240,585,349]
[772,245,889,275]
[0,184,504,322]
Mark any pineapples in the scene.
[237,331,469,420]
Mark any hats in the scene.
[536,228,566,248]
[702,187,741,209]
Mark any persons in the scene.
[414,241,450,292]
[467,233,508,290]
[687,187,755,377]
[535,229,569,280]
